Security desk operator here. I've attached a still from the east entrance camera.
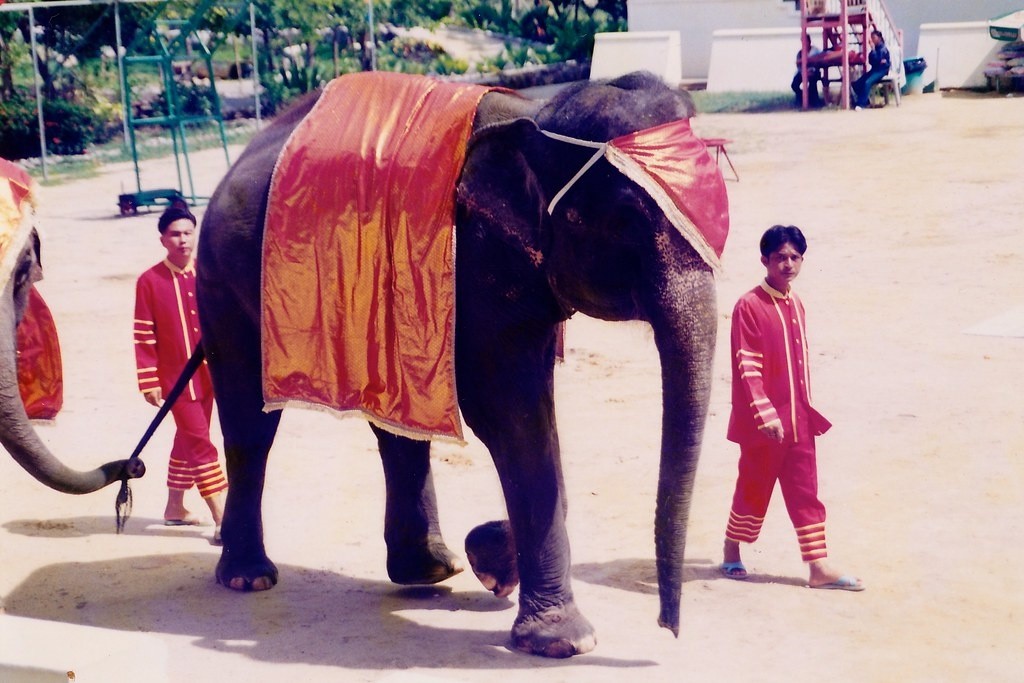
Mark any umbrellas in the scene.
[988,7,1024,43]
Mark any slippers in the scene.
[165,512,200,525]
[814,574,865,591]
[214,529,224,543]
[719,561,749,579]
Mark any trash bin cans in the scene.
[903,56,924,95]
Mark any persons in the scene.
[723,224,864,590]
[134,204,234,544]
[852,31,891,111]
[791,34,823,105]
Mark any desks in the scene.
[987,74,1024,93]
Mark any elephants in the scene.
[0,158,145,496]
[115,68,731,659]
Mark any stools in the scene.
[871,74,901,107]
